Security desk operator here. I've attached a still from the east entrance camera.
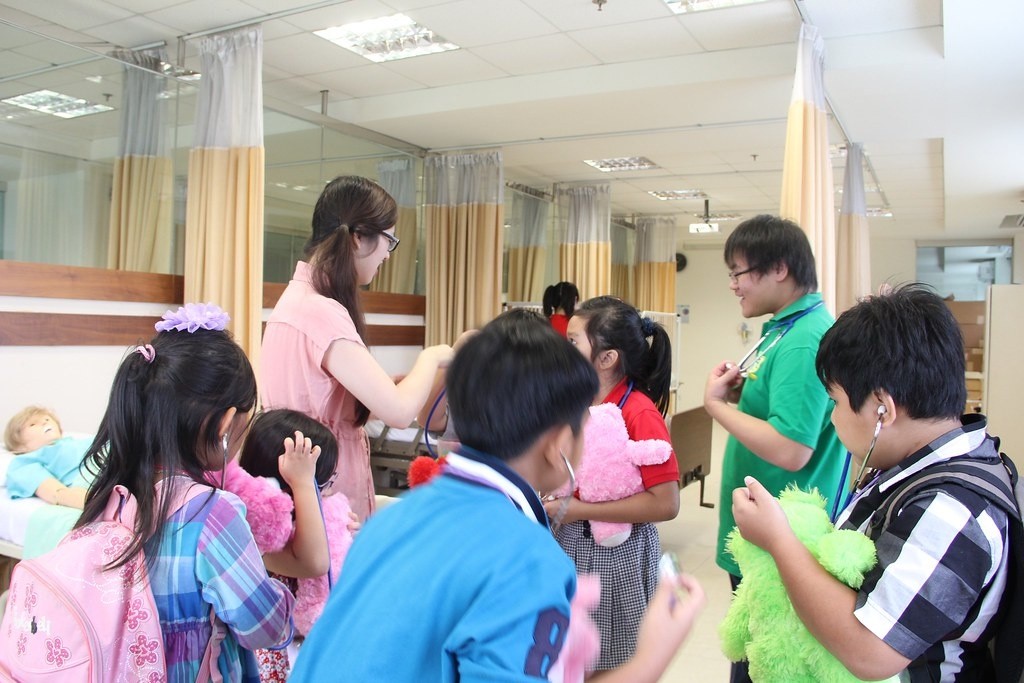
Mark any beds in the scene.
[361,405,716,511]
[0,445,82,563]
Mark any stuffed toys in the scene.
[717,483,901,683]
[549,402,671,548]
[203,461,294,553]
[555,572,602,683]
[292,492,356,638]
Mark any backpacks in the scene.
[0,476,224,683]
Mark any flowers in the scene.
[155,300,231,333]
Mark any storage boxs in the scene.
[940,293,985,414]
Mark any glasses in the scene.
[727,268,753,285]
[378,231,401,252]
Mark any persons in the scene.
[0,175,853,683]
[731,281,1024,683]
[4,405,110,511]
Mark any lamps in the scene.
[688,199,719,235]
